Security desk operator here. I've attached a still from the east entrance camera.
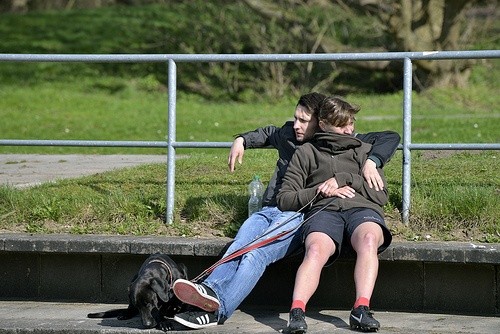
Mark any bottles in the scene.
[247,175,264,218]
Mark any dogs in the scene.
[87,255,190,333]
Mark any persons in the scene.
[169,92,405,330]
[278,98,387,333]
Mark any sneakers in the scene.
[174,311,217,329]
[288,308,307,334]
[172,278,220,312]
[349,306,380,332]
